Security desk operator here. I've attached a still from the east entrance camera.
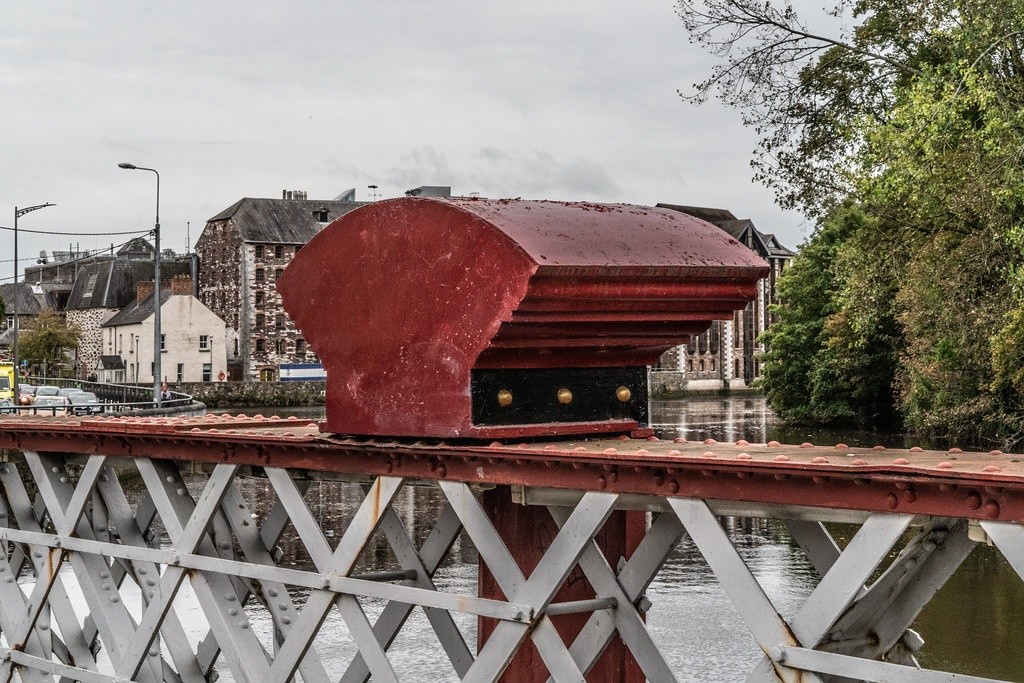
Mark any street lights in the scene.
[13,203,57,414]
[119,161,162,409]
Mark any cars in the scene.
[0,360,102,417]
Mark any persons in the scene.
[161,382,171,400]
